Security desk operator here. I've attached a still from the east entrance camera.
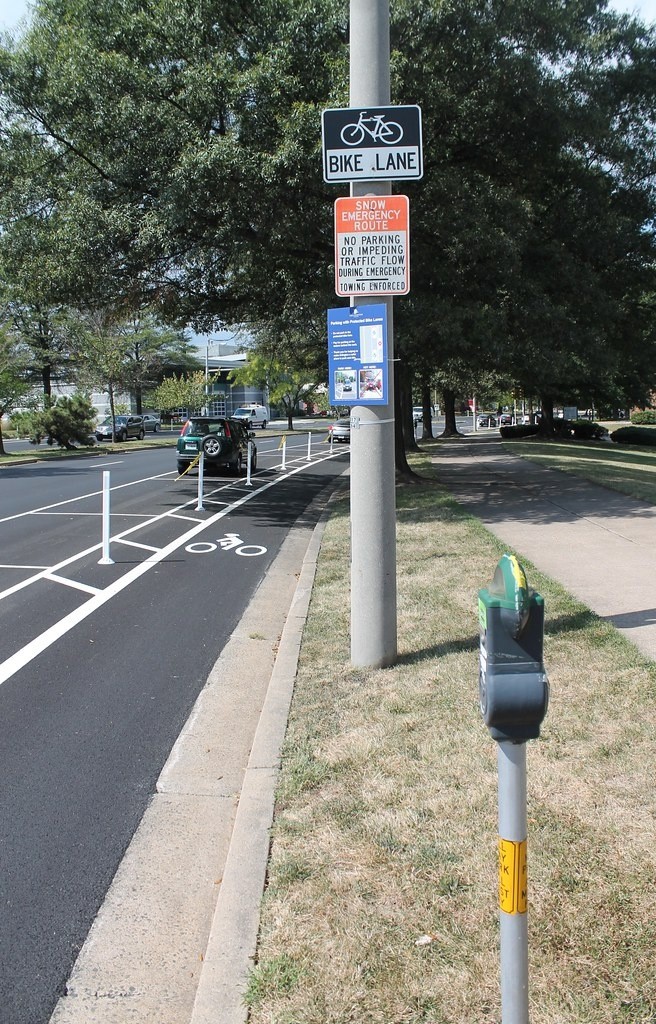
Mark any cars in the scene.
[477,415,497,426]
[521,414,529,422]
[328,418,350,443]
[498,413,518,426]
[413,414,417,428]
[532,411,542,419]
[140,415,161,432]
[413,407,434,421]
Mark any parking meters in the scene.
[476,555,550,1024]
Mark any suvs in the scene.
[231,404,269,429]
[94,415,145,442]
[175,416,257,475]
